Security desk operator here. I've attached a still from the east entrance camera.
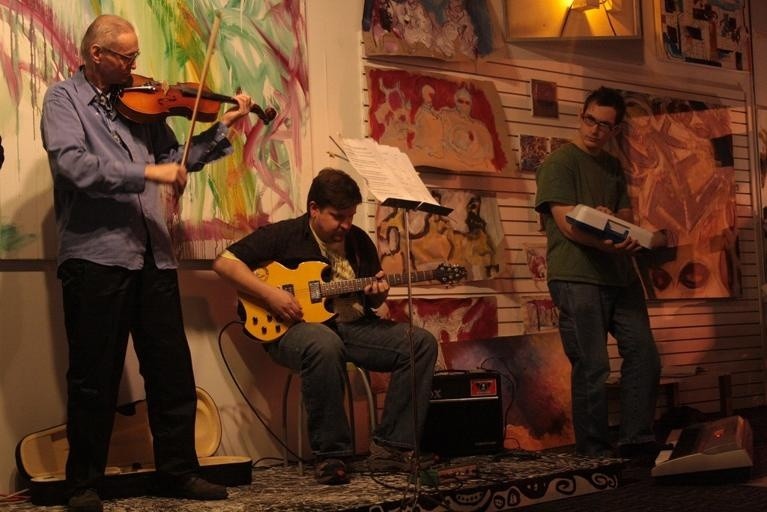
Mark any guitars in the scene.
[233,255,469,344]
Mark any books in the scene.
[334,130,440,207]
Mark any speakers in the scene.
[421,371,502,455]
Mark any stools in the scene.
[281,360,378,477]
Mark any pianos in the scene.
[648,413,757,488]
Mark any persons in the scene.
[531,85,668,459]
[458,194,501,278]
[437,86,496,171]
[41,12,255,511]
[208,164,440,484]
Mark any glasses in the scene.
[582,115,618,132]
[101,44,142,65]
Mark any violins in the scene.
[110,73,277,125]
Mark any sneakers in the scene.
[575,431,622,457]
[315,455,350,486]
[67,486,106,512]
[367,441,439,472]
[157,474,228,501]
